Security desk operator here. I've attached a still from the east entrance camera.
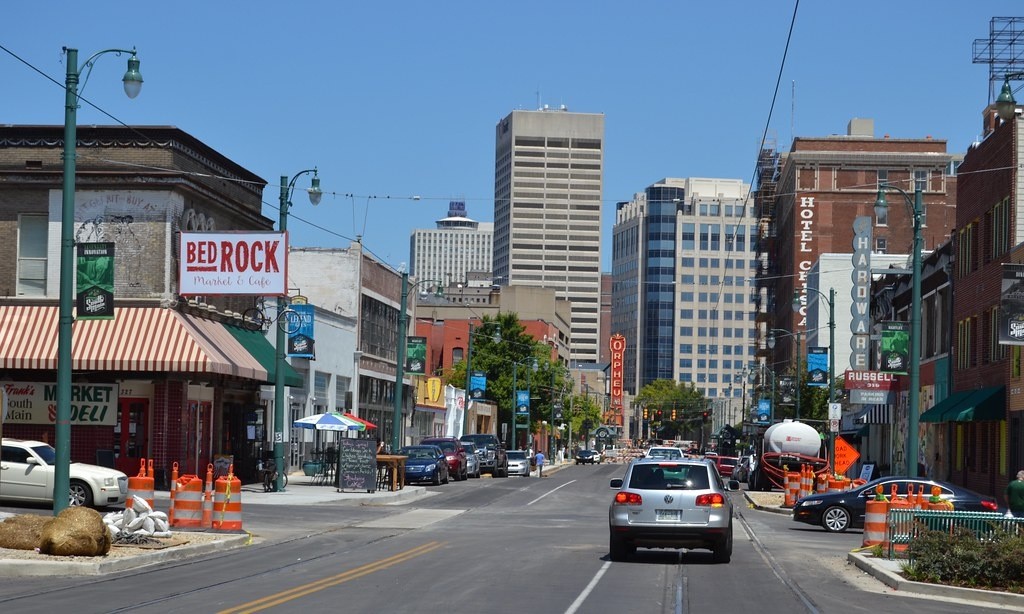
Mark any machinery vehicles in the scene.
[752,419,824,491]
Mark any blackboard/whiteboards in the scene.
[335,437,376,490]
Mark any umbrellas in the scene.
[291,412,378,482]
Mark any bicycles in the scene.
[256,455,288,493]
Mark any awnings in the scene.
[919,385,1006,424]
[223,323,303,389]
[853,404,895,425]
[0,303,268,383]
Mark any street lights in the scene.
[459,319,503,436]
[47,46,144,520]
[263,167,323,490]
[768,328,802,422]
[871,177,931,478]
[544,361,556,465]
[392,272,443,458]
[738,365,752,425]
[789,284,840,477]
[513,355,543,451]
[748,363,777,429]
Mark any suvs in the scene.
[459,434,511,481]
[607,457,741,564]
[416,435,470,480]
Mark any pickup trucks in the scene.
[704,454,758,484]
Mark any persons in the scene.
[535,451,545,478]
[376,439,386,468]
[558,445,569,461]
[518,446,533,458]
[688,445,700,454]
[1004,471,1024,517]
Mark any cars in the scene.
[459,440,482,478]
[399,444,449,485]
[792,475,1000,535]
[592,449,602,465]
[0,437,130,509]
[505,449,532,477]
[577,449,595,464]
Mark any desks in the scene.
[375,455,409,491]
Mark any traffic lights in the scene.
[657,410,662,421]
[703,411,709,424]
